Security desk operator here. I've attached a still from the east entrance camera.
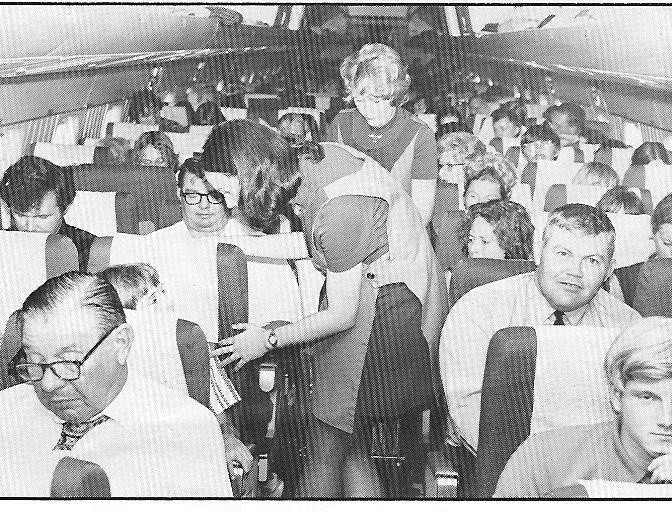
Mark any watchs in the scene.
[268,329,278,347]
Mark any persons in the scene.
[0,155,97,271]
[596,186,644,216]
[102,264,254,480]
[200,118,454,503]
[435,131,487,183]
[543,103,590,144]
[520,124,560,164]
[170,56,328,124]
[0,271,234,502]
[412,57,535,137]
[491,316,672,503]
[438,204,641,458]
[96,137,132,166]
[463,153,518,207]
[630,141,668,166]
[328,44,439,232]
[133,131,179,169]
[148,154,266,241]
[127,90,187,135]
[615,193,672,306]
[455,198,535,262]
[572,161,620,203]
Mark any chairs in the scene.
[624,163,672,219]
[521,161,605,208]
[0,230,79,309]
[64,188,136,237]
[267,108,323,139]
[103,122,164,143]
[85,235,295,499]
[476,325,624,503]
[0,308,206,416]
[434,211,471,274]
[449,258,537,311]
[617,258,672,319]
[504,146,593,177]
[79,139,136,160]
[159,106,188,128]
[306,95,344,126]
[27,141,115,175]
[593,147,640,182]
[545,184,650,217]
[245,93,281,123]
[68,162,182,237]
[221,105,251,127]
[435,184,460,234]
[163,130,208,165]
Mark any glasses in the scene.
[181,192,226,204]
[9,325,119,382]
